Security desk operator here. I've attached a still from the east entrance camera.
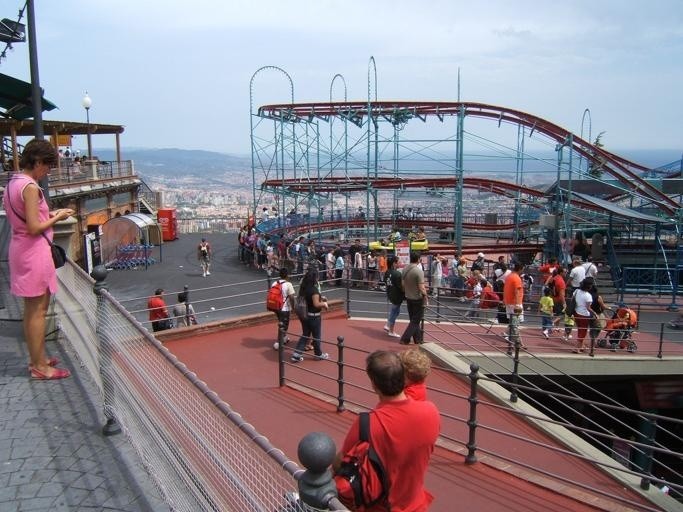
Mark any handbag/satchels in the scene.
[158,315,174,331]
[51,243,67,270]
[593,312,607,329]
[496,303,510,324]
[565,290,578,317]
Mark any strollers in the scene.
[597,306,638,352]
[520,273,534,311]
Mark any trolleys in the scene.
[109,243,156,270]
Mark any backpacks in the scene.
[266,279,290,313]
[293,295,309,321]
[547,277,559,297]
[199,242,209,258]
[332,410,394,512]
[487,291,500,309]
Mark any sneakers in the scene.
[282,335,291,345]
[304,338,314,351]
[26,356,62,373]
[272,341,280,352]
[541,321,572,343]
[312,352,331,361]
[388,330,401,338]
[290,351,305,362]
[502,331,527,351]
[29,365,72,381]
[383,323,390,333]
[576,347,585,354]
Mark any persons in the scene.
[290,269,330,361]
[270,267,296,350]
[174,295,198,328]
[334,350,440,511]
[60,146,87,177]
[399,251,429,344]
[299,277,327,351]
[538,230,631,353]
[397,350,432,400]
[198,237,213,277]
[382,256,404,337]
[240,204,533,324]
[3,139,75,379]
[147,289,172,332]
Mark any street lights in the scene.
[82,89,94,162]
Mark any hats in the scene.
[476,252,486,258]
